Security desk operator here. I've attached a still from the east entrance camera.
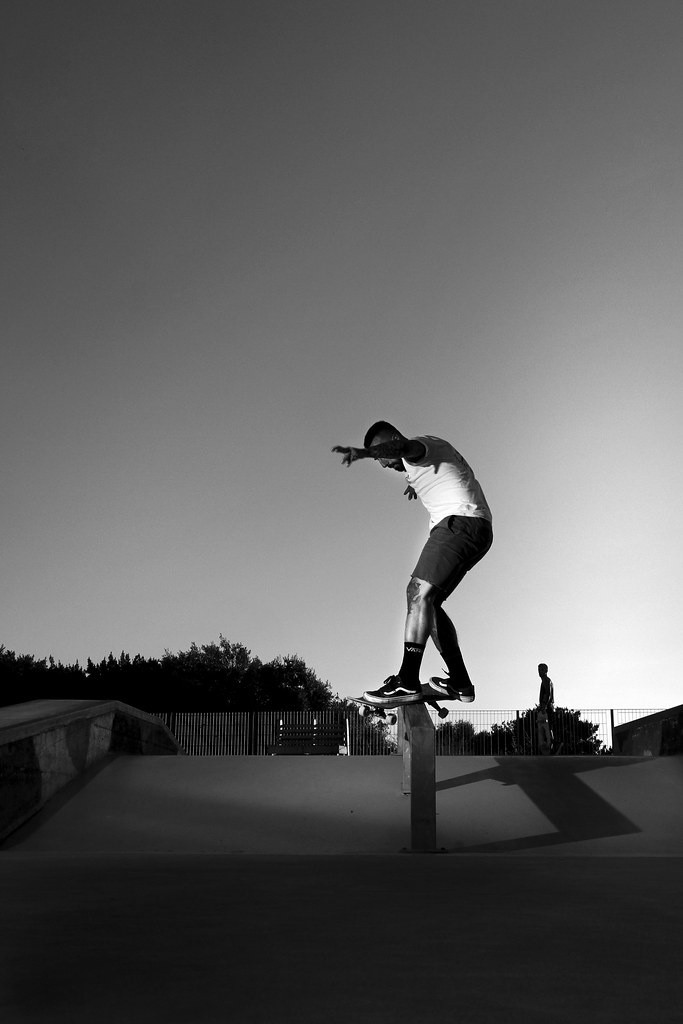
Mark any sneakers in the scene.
[429,677,475,703]
[363,675,423,703]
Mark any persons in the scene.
[537,664,560,754]
[331,421,493,704]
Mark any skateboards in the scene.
[347,682,456,726]
